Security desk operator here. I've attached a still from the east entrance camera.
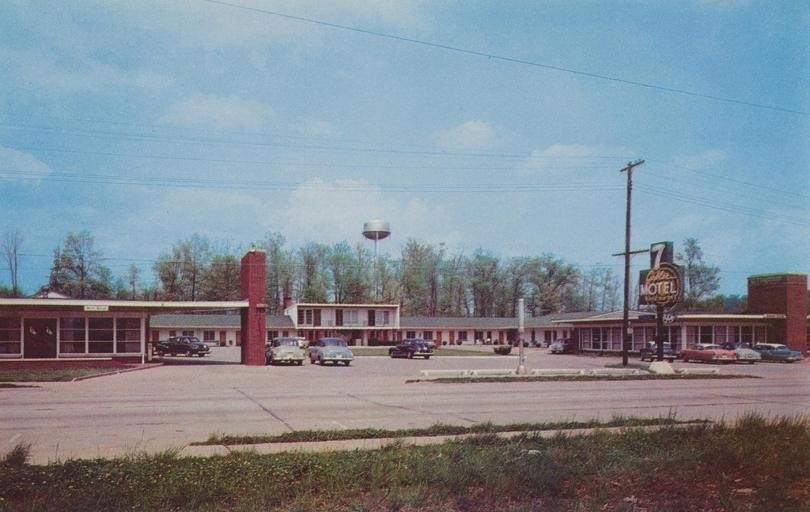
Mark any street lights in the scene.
[516,297,529,375]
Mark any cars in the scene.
[749,341,803,364]
[386,338,434,360]
[547,337,571,354]
[716,339,763,365]
[308,337,354,367]
[263,336,306,367]
[637,340,678,364]
[678,342,737,364]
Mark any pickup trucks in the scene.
[155,335,211,358]
